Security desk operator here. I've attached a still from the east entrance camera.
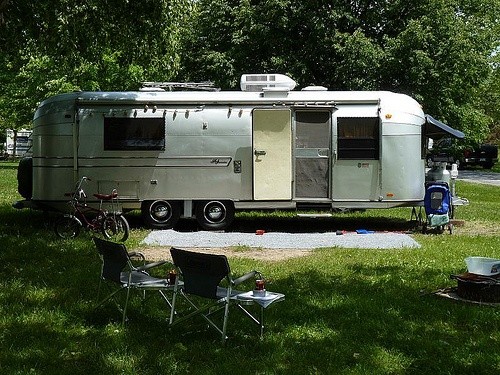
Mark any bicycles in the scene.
[55,177,130,243]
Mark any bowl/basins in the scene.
[253,289,267,297]
[256,230,265,235]
[463,257,500,276]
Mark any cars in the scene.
[425,138,498,170]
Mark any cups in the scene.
[167,273,176,285]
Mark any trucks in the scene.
[13,74,465,231]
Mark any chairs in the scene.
[93,235,185,329]
[169,246,285,344]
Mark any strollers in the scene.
[422,181,454,235]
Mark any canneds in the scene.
[256,230,264,235]
[255,279,264,290]
